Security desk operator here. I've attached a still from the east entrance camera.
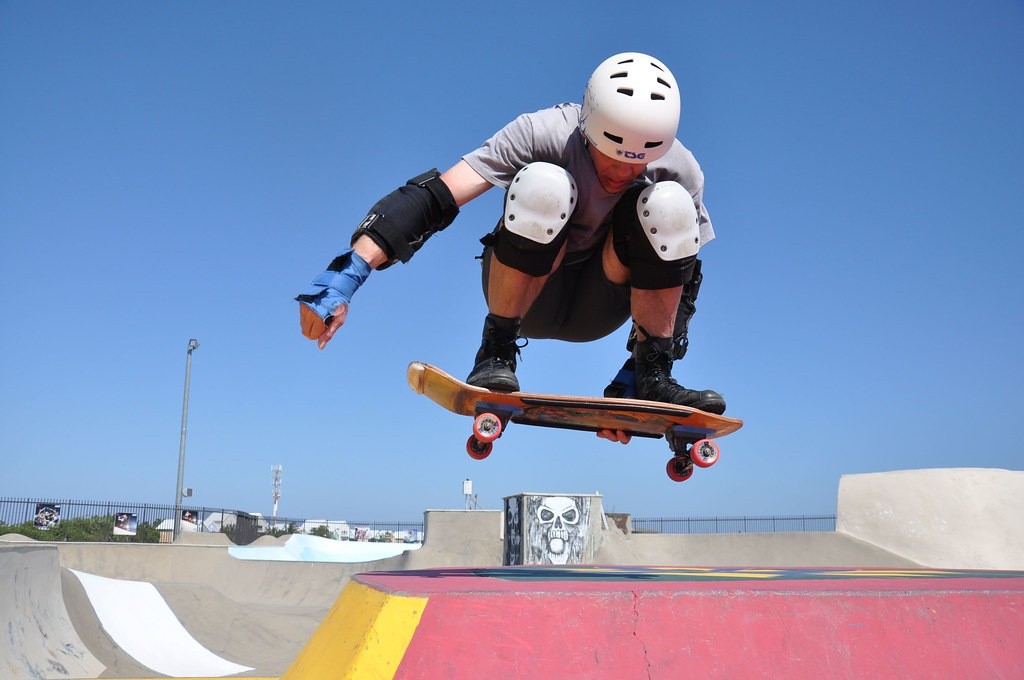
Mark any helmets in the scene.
[580,53,680,164]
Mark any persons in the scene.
[293,53,726,445]
[355,527,368,539]
[35,507,59,526]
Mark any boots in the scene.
[466,314,528,391]
[632,319,726,416]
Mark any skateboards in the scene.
[406,360,748,483]
